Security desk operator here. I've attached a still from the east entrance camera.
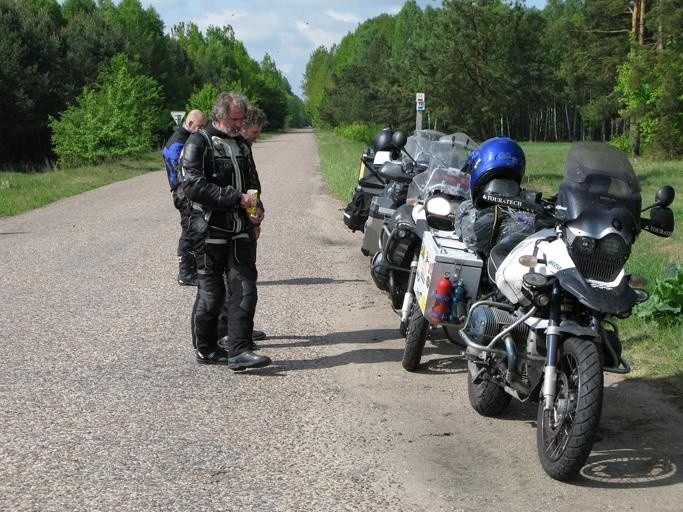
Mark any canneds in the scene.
[246,189,258,217]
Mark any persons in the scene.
[161,109,206,287]
[178,90,271,372]
[239,105,266,349]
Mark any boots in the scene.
[175,256,201,286]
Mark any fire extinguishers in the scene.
[447,279,467,323]
[432,272,451,323]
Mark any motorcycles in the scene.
[457,139,678,480]
[349,124,502,374]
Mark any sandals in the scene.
[194,344,231,366]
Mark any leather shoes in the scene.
[225,350,274,371]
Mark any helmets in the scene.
[369,129,404,161]
[460,134,530,210]
